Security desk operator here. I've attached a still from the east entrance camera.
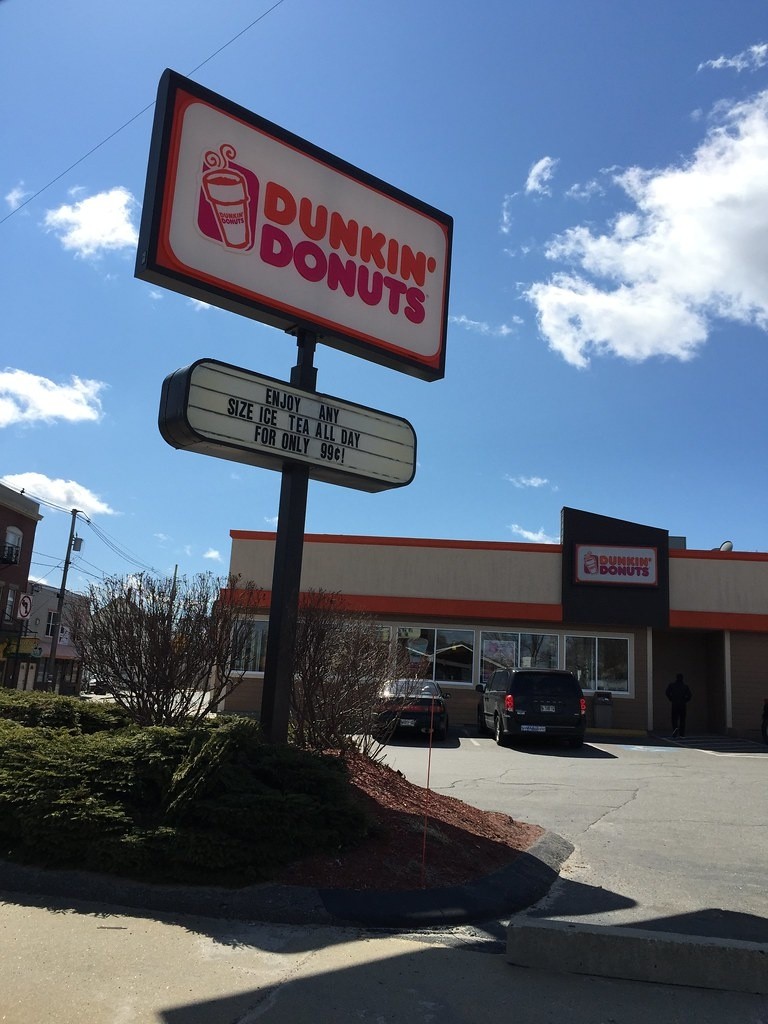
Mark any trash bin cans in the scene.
[593,691,614,728]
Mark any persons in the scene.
[760,698,768,743]
[665,673,692,740]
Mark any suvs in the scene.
[475,666,588,750]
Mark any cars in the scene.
[81,672,107,695]
[369,677,453,745]
